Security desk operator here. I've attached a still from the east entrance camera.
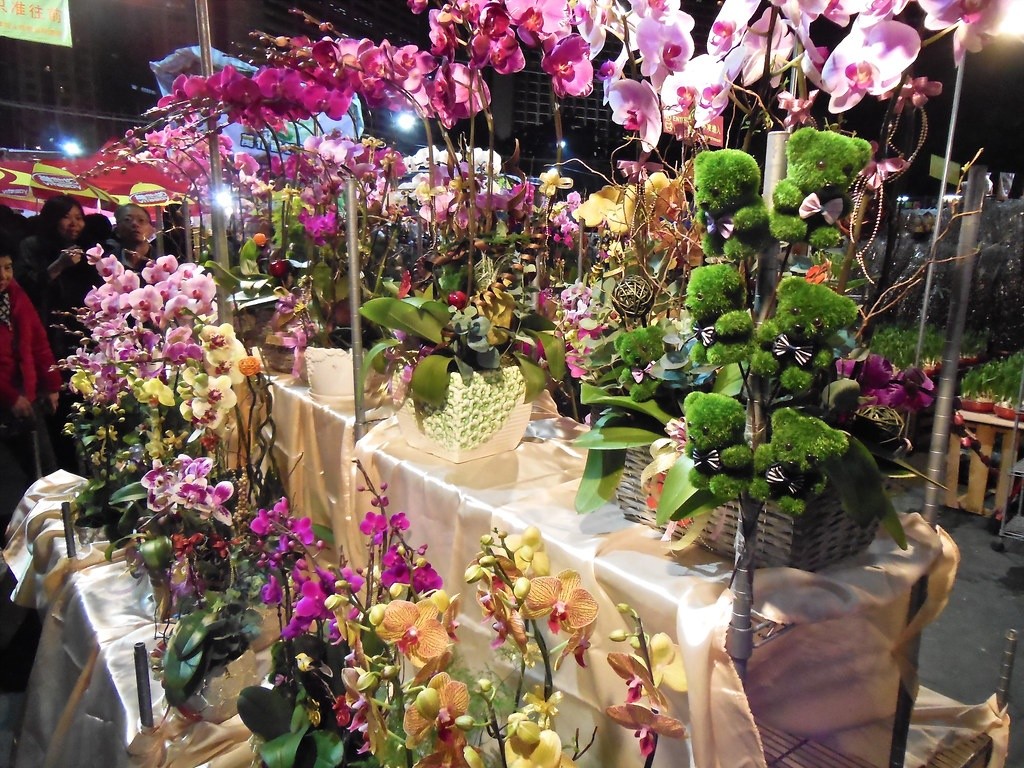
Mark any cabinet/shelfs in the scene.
[6,346,1010,768]
[991,366,1024,552]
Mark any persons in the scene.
[0,231,64,417]
[0,196,187,429]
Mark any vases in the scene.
[201,583,224,613]
[178,645,260,726]
[304,346,374,402]
[149,570,180,621]
[252,304,330,372]
[73,513,113,546]
[389,355,534,463]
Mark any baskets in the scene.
[617,451,885,573]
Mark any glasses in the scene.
[115,215,152,225]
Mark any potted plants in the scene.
[961,388,1024,421]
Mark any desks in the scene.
[945,409,1024,522]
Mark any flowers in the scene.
[45,0,1009,768]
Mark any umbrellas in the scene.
[0,143,256,217]
